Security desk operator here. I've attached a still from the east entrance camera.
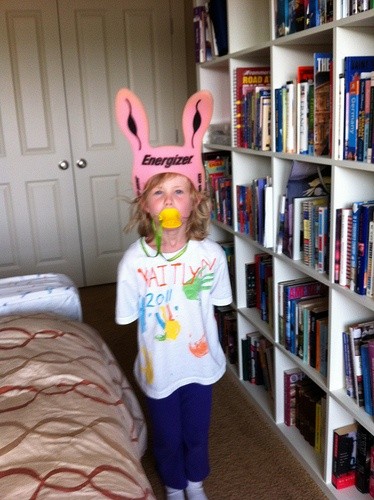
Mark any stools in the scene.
[0,274,82,320]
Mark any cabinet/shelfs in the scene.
[194,0,374,500]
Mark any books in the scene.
[275,0,374,38]
[233,52,374,164]
[284,368,327,465]
[214,305,238,365]
[202,150,233,227]
[278,276,328,377]
[241,331,274,401]
[342,320,374,416]
[245,252,273,329]
[236,175,374,298]
[192,6,213,63]
[216,239,236,302]
[331,422,374,498]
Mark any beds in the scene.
[0,312,157,500]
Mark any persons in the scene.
[115,147,233,500]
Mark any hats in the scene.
[114,88,213,198]
